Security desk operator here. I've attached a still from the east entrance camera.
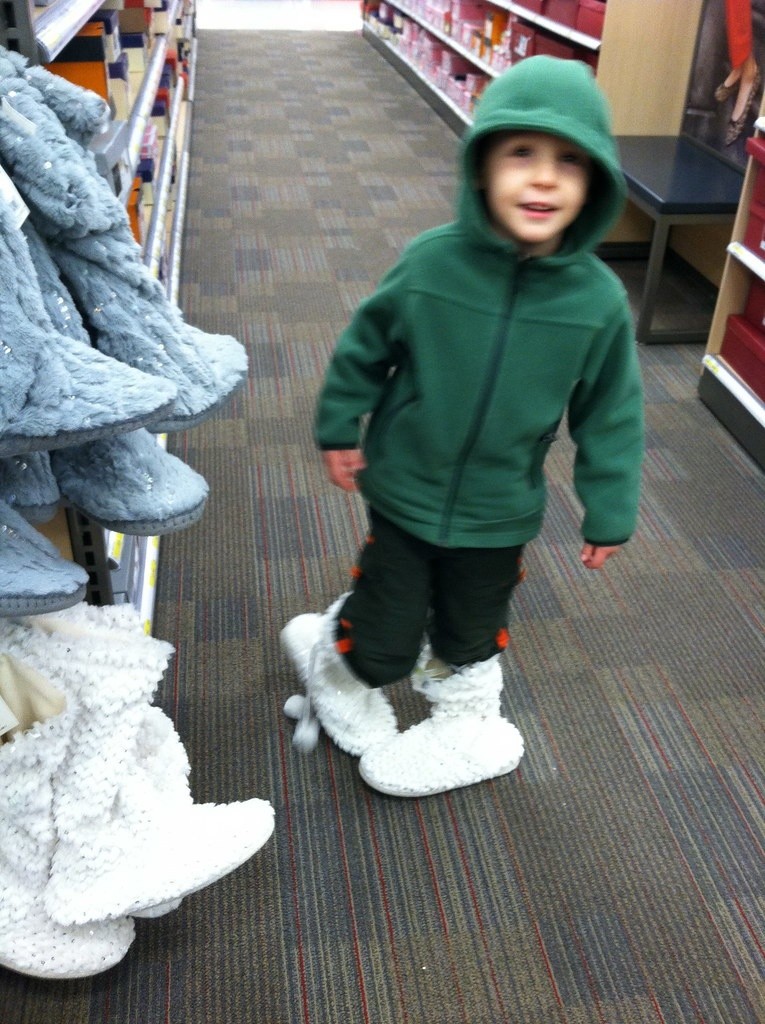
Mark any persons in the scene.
[274,54,646,797]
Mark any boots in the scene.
[0,606,276,980]
[280,591,399,758]
[359,645,525,798]
[0,43,249,618]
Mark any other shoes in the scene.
[726,68,762,146]
[714,69,742,103]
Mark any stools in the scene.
[615,136,748,337]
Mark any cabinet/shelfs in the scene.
[360,1,605,136]
[0,0,198,634]
[698,140,765,475]
